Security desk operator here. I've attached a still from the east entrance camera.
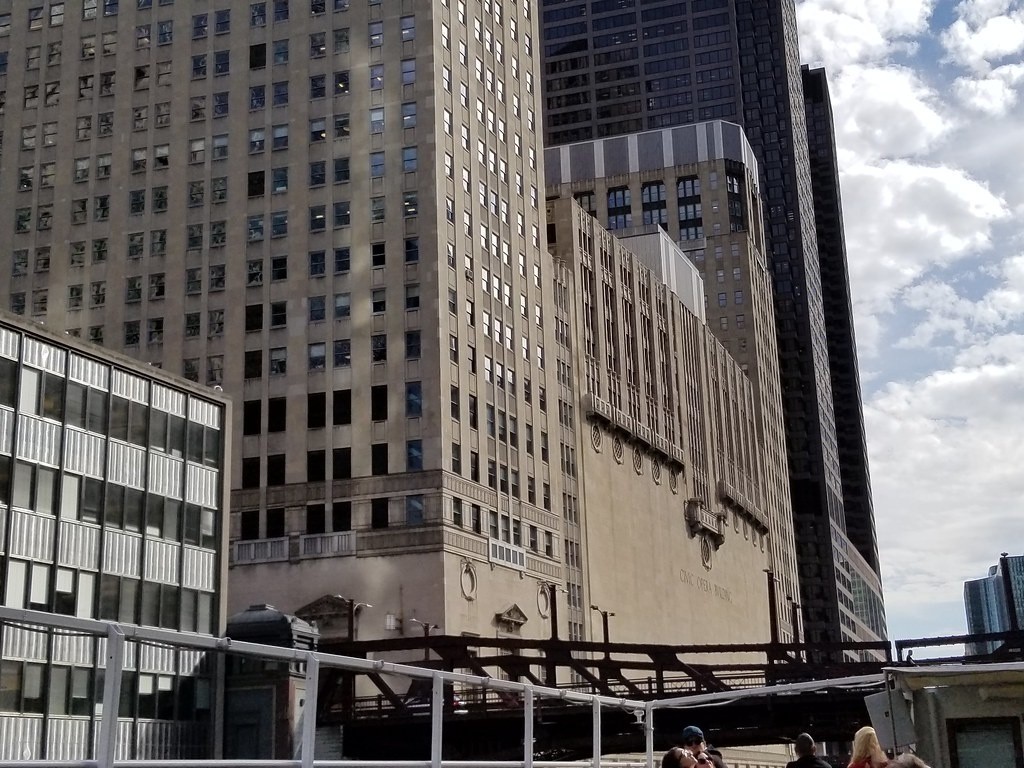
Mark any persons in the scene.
[786,733,831,768]
[662,725,728,768]
[846,726,893,768]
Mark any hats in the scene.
[682,726,703,738]
[796,733,814,753]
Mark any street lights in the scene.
[763,567,781,664]
[786,594,803,663]
[408,617,441,660]
[590,604,615,658]
[334,593,374,725]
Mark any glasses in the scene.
[679,749,689,761]
[698,757,713,764]
[685,738,703,747]
